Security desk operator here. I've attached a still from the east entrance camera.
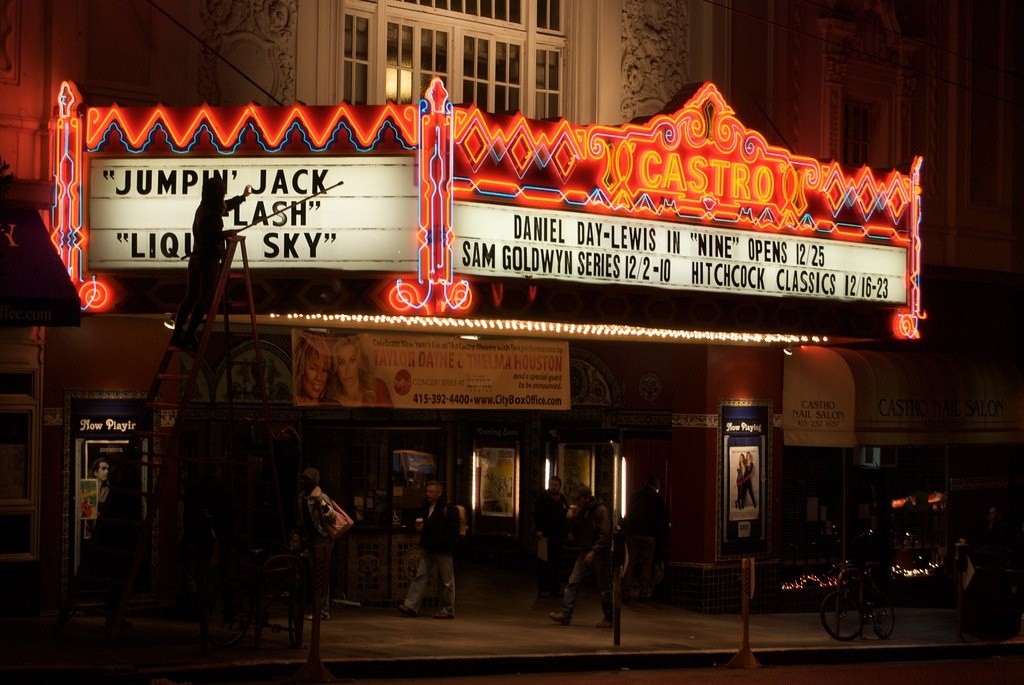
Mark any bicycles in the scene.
[819,561,896,642]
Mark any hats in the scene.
[301,467,320,483]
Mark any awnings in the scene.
[0,208,82,329]
[783,346,1024,447]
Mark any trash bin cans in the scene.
[955,565,1023,642]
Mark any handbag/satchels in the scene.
[314,494,355,539]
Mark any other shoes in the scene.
[307,610,331,620]
[398,605,416,616]
[596,617,614,630]
[549,611,572,625]
[329,611,340,621]
[432,608,454,618]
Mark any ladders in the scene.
[51,233,275,651]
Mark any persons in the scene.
[735,452,757,509]
[534,476,573,599]
[549,486,614,629]
[399,481,460,619]
[981,506,1009,562]
[169,178,251,346]
[242,416,302,548]
[293,330,393,406]
[92,458,110,502]
[297,467,335,620]
